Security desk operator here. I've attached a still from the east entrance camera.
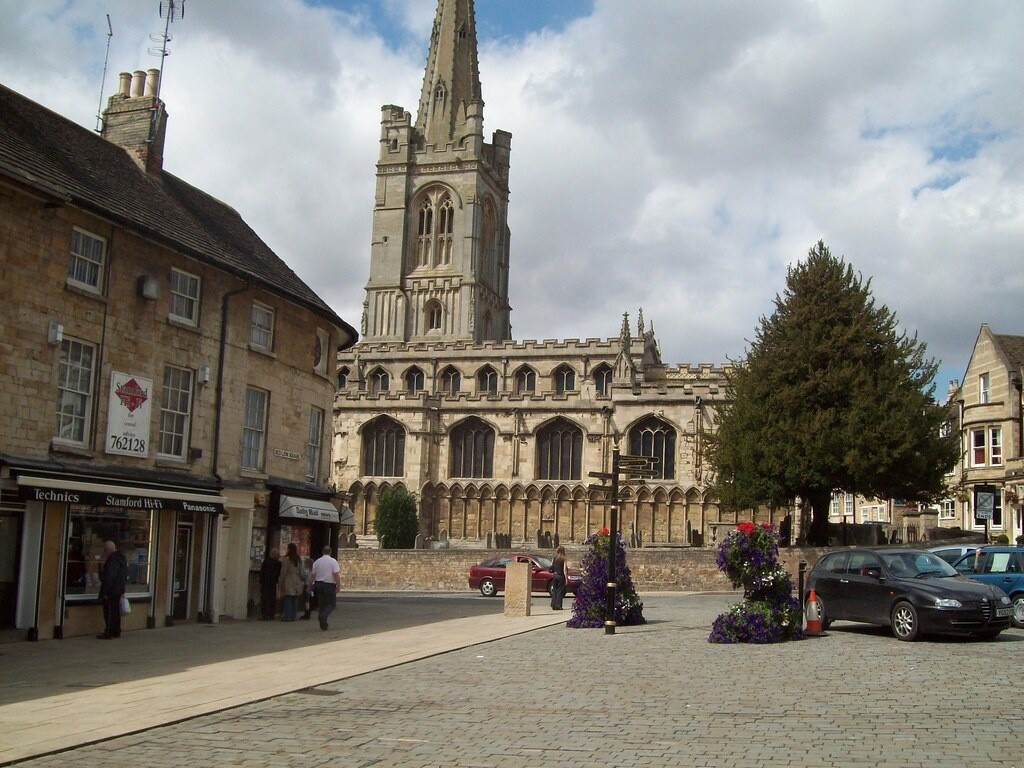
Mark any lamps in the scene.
[47,320,63,345]
[431,360,437,365]
[581,356,588,362]
[501,359,508,364]
[197,365,210,385]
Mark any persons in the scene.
[347,533,358,548]
[550,546,568,610]
[300,558,316,620]
[973,548,982,569]
[96,541,128,640]
[338,532,347,548]
[310,546,341,631]
[258,547,282,620]
[279,543,306,621]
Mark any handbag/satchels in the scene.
[120,596,131,615]
[548,566,554,573]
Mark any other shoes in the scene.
[281,616,294,622]
[112,632,120,638]
[97,634,112,640]
[258,616,268,620]
[319,615,328,630]
[300,615,311,620]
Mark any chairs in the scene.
[830,559,846,573]
[890,560,906,577]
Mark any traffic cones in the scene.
[801,589,829,636]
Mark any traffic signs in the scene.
[589,471,613,479]
[616,492,632,499]
[620,454,660,464]
[617,461,647,467]
[618,467,659,477]
[587,484,613,491]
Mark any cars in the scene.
[912,543,991,580]
[949,546,1024,629]
[469,554,586,599]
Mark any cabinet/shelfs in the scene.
[68,536,151,566]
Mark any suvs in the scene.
[804,546,1015,642]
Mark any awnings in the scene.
[279,495,340,522]
[340,506,357,526]
[11,469,229,515]
